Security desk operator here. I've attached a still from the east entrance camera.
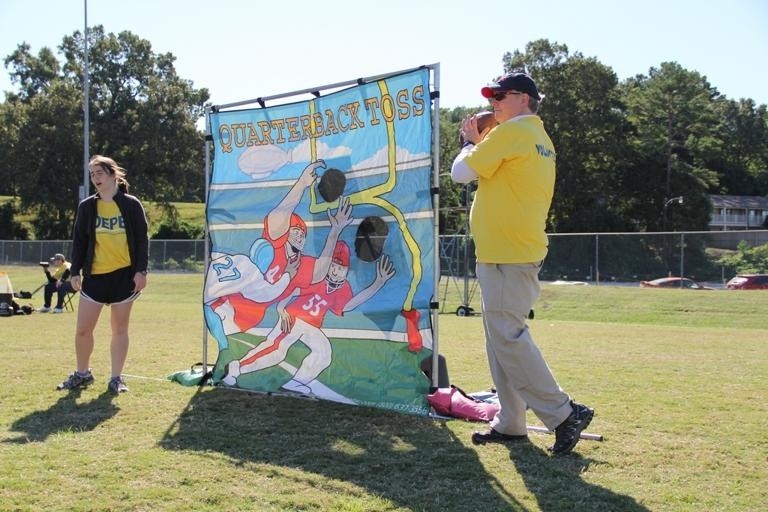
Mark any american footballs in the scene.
[318,167,346,202]
[459,112,496,145]
[354,216,389,262]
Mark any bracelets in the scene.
[141,271,147,275]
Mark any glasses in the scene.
[490,91,521,100]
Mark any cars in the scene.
[727,274,768,289]
[640,277,715,290]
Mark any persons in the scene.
[38,253,77,313]
[201,239,302,374]
[219,239,397,395]
[209,159,354,338]
[56,155,148,395]
[447,71,596,453]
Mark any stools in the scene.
[63,290,77,312]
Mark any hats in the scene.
[49,253,65,262]
[480,72,542,103]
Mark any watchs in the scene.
[463,140,475,147]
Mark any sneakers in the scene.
[471,426,528,447]
[52,308,64,314]
[55,366,96,392]
[107,375,130,395]
[549,400,595,459]
[36,306,49,313]
